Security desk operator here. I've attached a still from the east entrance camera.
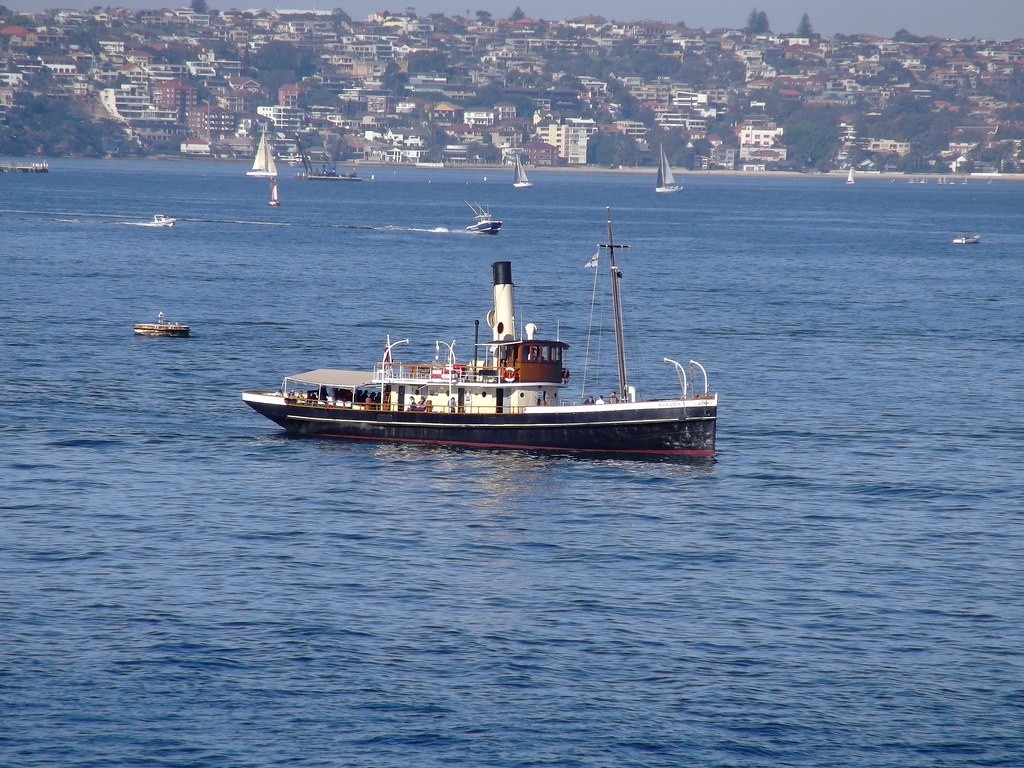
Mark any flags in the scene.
[584,251,600,268]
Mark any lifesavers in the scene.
[503,366,516,382]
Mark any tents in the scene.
[282,366,379,391]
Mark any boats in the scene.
[152,215,175,227]
[294,132,364,182]
[0,159,51,174]
[239,207,720,465]
[465,198,503,234]
[952,234,983,244]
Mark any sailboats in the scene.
[267,182,282,206]
[246,130,280,176]
[654,141,686,192]
[845,167,855,185]
[512,154,533,187]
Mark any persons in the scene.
[274,386,619,436]
